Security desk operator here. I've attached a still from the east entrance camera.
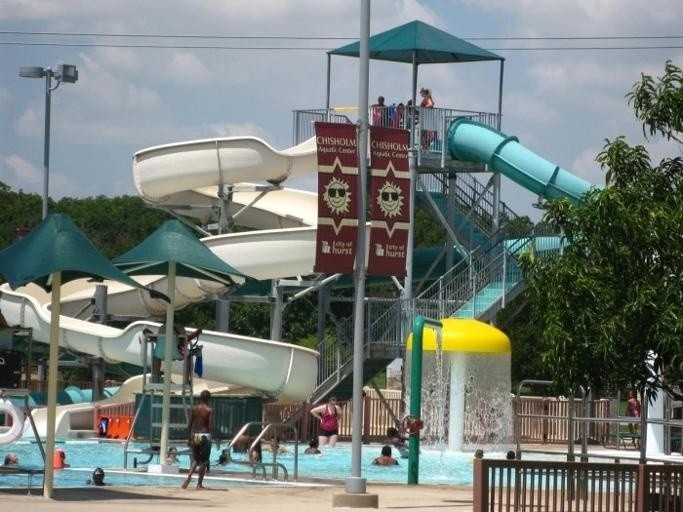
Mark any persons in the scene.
[245,438,261,466]
[309,396,342,446]
[506,450,515,459]
[268,434,288,454]
[467,448,483,465]
[3,452,18,464]
[150,312,186,382]
[624,389,641,447]
[371,445,398,465]
[180,389,214,488]
[386,427,410,458]
[209,450,228,467]
[85,467,111,486]
[303,436,321,454]
[165,446,178,462]
[369,84,436,153]
[231,422,250,451]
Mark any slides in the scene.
[0,119,605,438]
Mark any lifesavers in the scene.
[0,399,25,445]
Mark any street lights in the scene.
[17,62,79,393]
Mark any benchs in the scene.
[0,466,45,496]
[603,432,680,451]
